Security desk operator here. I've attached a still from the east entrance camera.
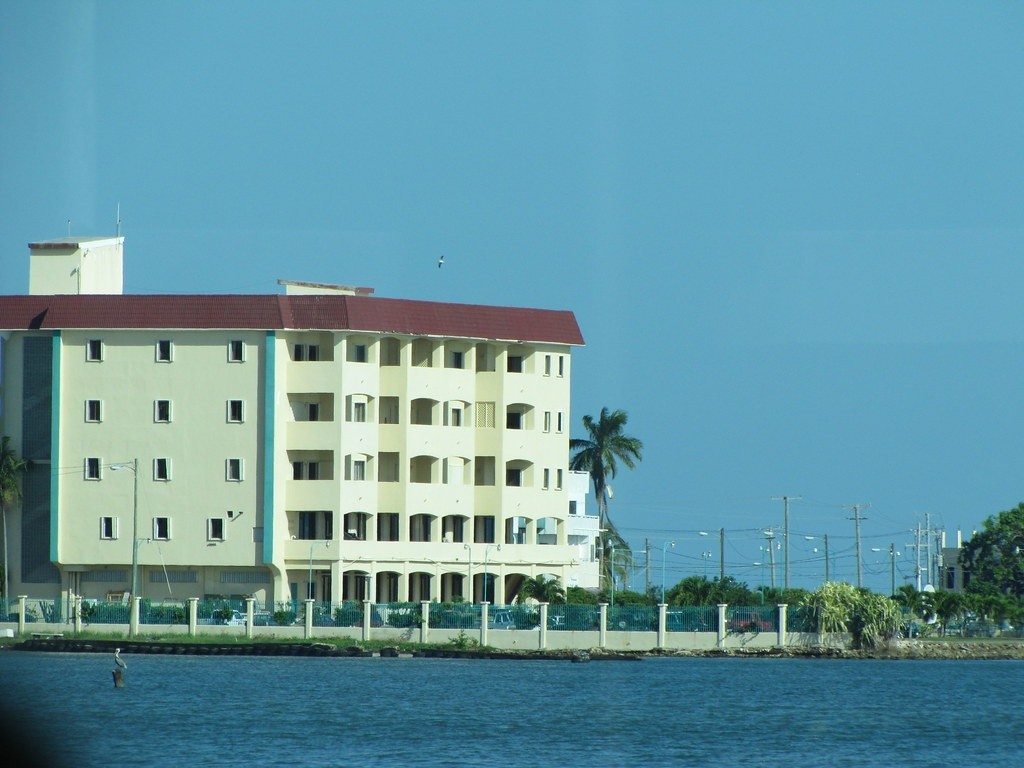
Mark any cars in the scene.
[550,616,567,625]
[904,623,919,638]
[494,612,516,629]
[965,619,1024,638]
[613,607,773,632]
[211,609,246,626]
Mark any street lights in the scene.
[701,551,712,577]
[699,527,724,580]
[804,534,829,584]
[109,458,141,601]
[753,528,787,603]
[611,547,646,607]
[872,543,897,599]
[464,544,473,604]
[484,544,503,601]
[309,540,332,598]
[661,540,676,604]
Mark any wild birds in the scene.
[114,648,127,671]
[439,256,444,267]
